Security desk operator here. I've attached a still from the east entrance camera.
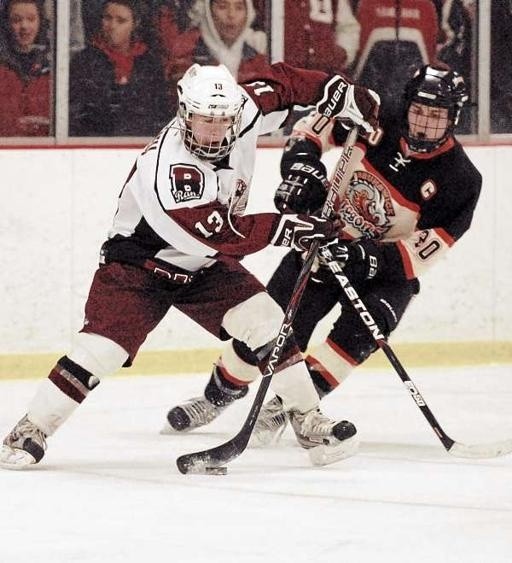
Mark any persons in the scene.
[1,1,271,136]
[286,1,512,129]
[165,60,481,447]
[3,61,382,467]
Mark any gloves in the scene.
[316,75,381,138]
[301,236,383,283]
[273,156,331,215]
[267,212,346,250]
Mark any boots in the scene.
[168,365,246,431]
[4,410,51,464]
[251,363,357,449]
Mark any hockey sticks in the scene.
[177,126,359,475]
[318,246,512,460]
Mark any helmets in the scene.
[399,62,468,160]
[177,62,244,164]
